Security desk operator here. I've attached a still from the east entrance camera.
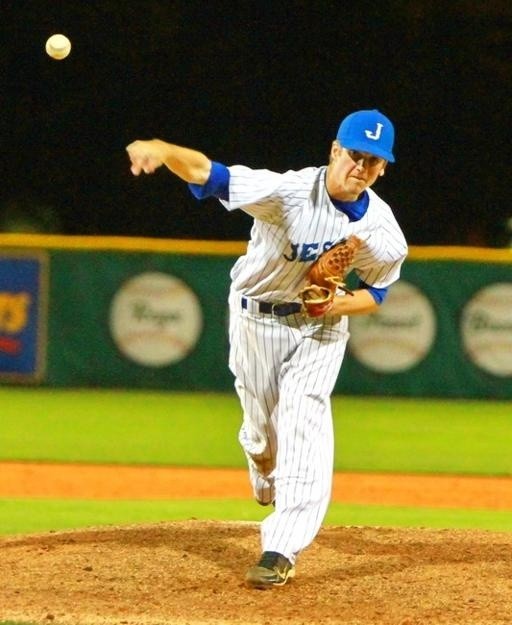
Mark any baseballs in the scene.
[46,34,71,60]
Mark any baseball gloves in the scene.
[299,235,362,318]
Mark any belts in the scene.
[241,299,302,316]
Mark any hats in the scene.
[336,110,395,163]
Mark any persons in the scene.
[126,104,413,591]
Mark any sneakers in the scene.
[245,551,296,587]
